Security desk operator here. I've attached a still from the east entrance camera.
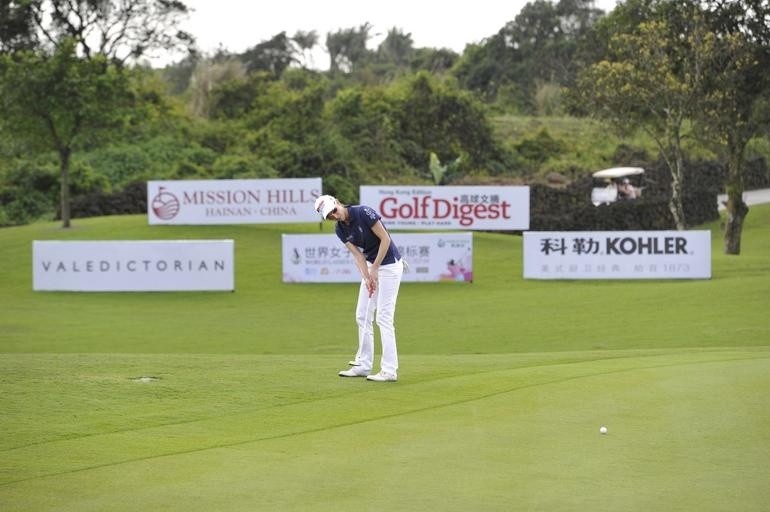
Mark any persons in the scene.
[618,178,637,200]
[603,177,617,190]
[313,194,403,383]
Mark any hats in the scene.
[314,194,336,222]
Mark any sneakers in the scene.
[366,371,398,382]
[338,366,372,377]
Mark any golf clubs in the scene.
[349,287,374,366]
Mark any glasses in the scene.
[326,208,337,219]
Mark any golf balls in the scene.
[600,426,607,434]
